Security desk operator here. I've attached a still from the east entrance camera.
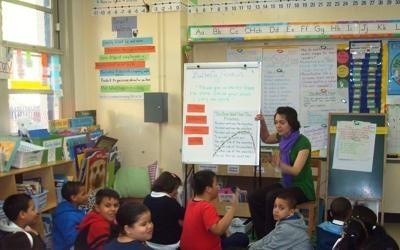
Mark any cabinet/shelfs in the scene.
[0,159,77,250]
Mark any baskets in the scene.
[11,141,49,169]
[32,190,49,211]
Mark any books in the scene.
[27,116,104,164]
[16,177,43,195]
[55,174,73,203]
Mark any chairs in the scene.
[292,160,322,240]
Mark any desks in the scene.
[212,201,255,239]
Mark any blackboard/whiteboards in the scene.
[180,60,263,167]
[324,112,387,202]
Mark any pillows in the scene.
[112,162,159,210]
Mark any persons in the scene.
[314,196,397,249]
[248,107,314,239]
[143,170,238,249]
[249,194,310,249]
[1,193,48,249]
[50,181,153,249]
